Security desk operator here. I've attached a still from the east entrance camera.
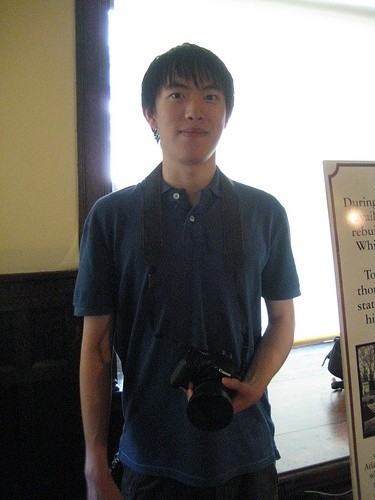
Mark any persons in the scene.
[71,44,301,500]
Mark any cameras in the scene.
[170,349,244,431]
